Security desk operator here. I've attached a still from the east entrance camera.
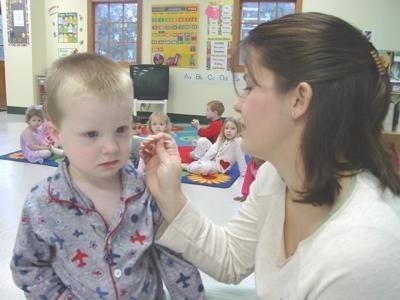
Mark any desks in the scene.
[134,99,167,116]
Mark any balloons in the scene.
[206,0,233,7]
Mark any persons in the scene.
[137,9,400,300]
[9,51,208,300]
[182,116,248,179]
[190,99,226,144]
[39,105,61,149]
[137,108,173,177]
[19,104,54,164]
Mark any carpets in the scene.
[0,126,248,188]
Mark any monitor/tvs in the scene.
[130,64,169,100]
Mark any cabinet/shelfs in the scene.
[36,74,48,111]
